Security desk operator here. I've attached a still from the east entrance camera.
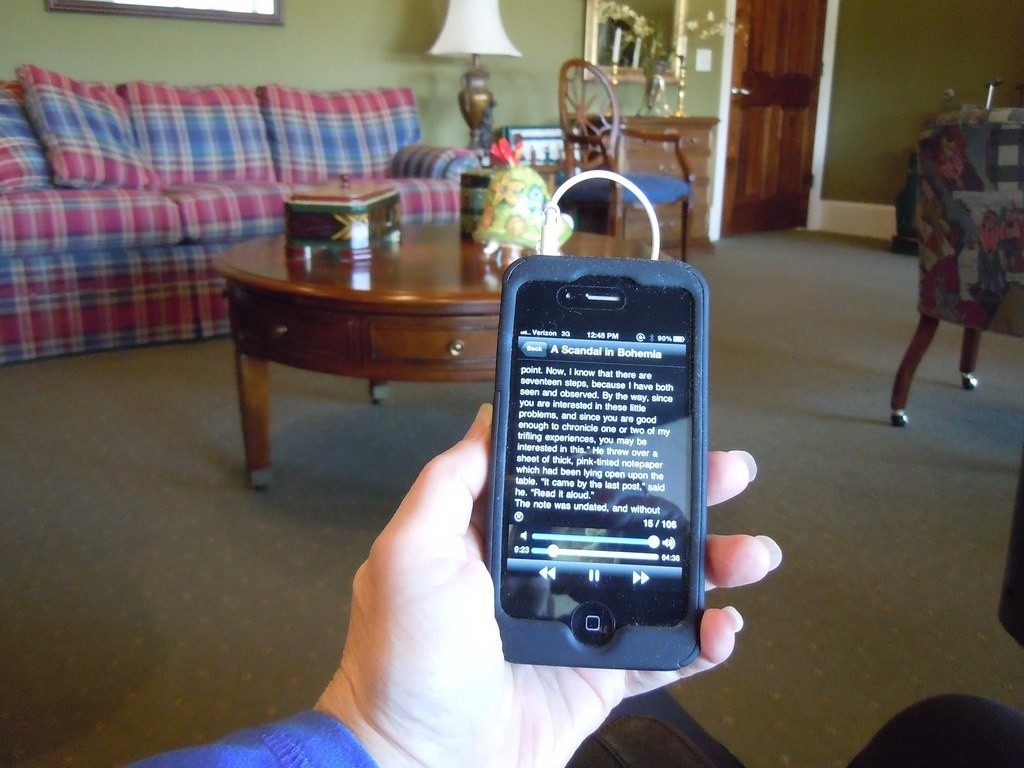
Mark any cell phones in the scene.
[485,255,710,669]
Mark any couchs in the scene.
[0,79,483,366]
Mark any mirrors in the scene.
[583,0,689,85]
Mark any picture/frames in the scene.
[46,1,285,28]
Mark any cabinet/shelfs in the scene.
[565,115,721,257]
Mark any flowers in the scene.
[595,3,749,74]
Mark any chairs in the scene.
[889,107,1024,430]
[558,59,692,264]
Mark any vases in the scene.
[636,68,676,119]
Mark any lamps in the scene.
[428,0,524,159]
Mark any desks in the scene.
[210,225,676,486]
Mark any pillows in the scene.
[17,64,161,191]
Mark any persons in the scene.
[122,399,1023,768]
